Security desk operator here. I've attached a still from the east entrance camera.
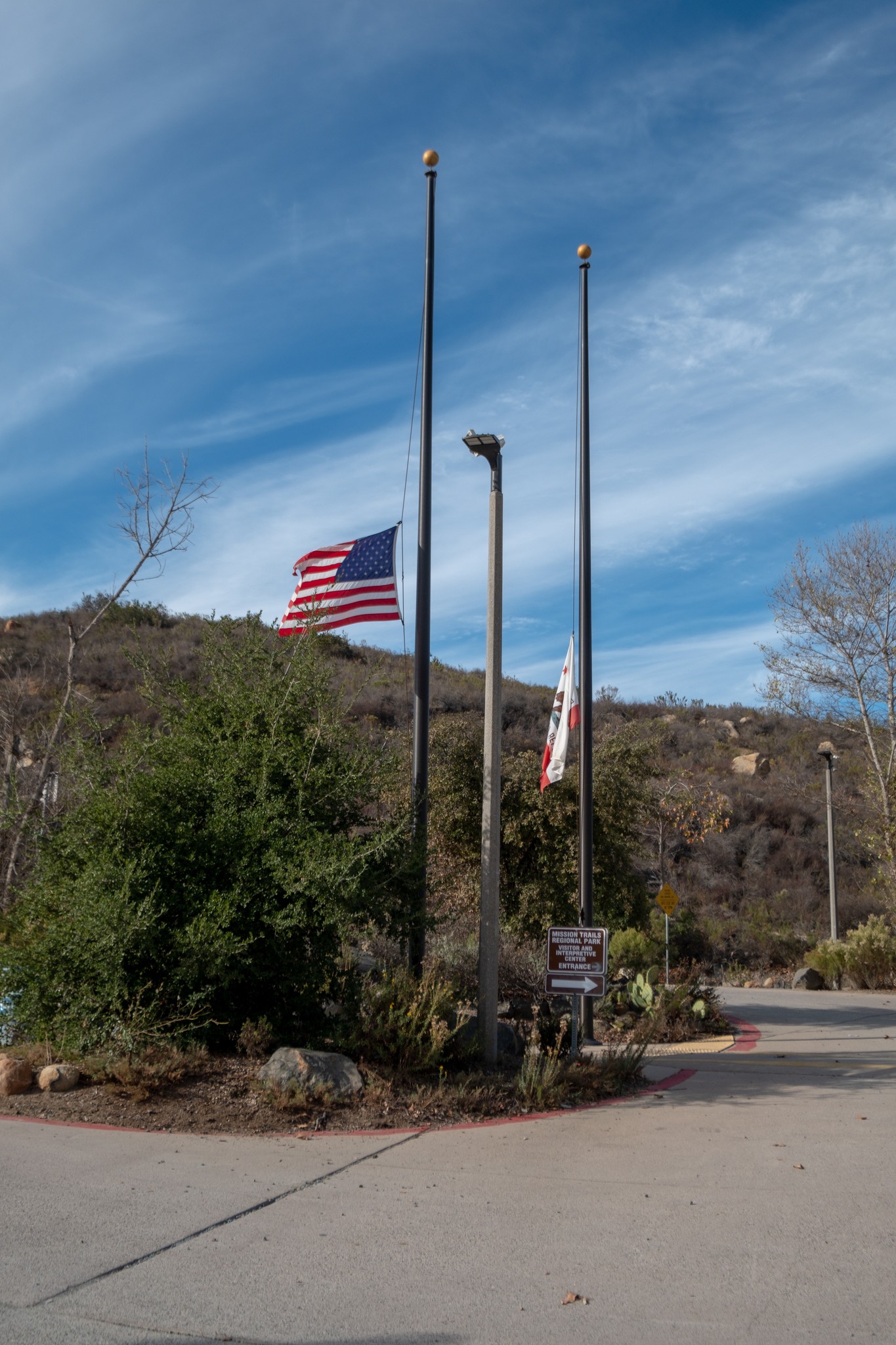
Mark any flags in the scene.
[278,526,402,638]
[541,635,582,793]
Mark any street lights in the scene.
[462,430,506,1075]
[817,750,838,943]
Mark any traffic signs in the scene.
[545,974,605,998]
